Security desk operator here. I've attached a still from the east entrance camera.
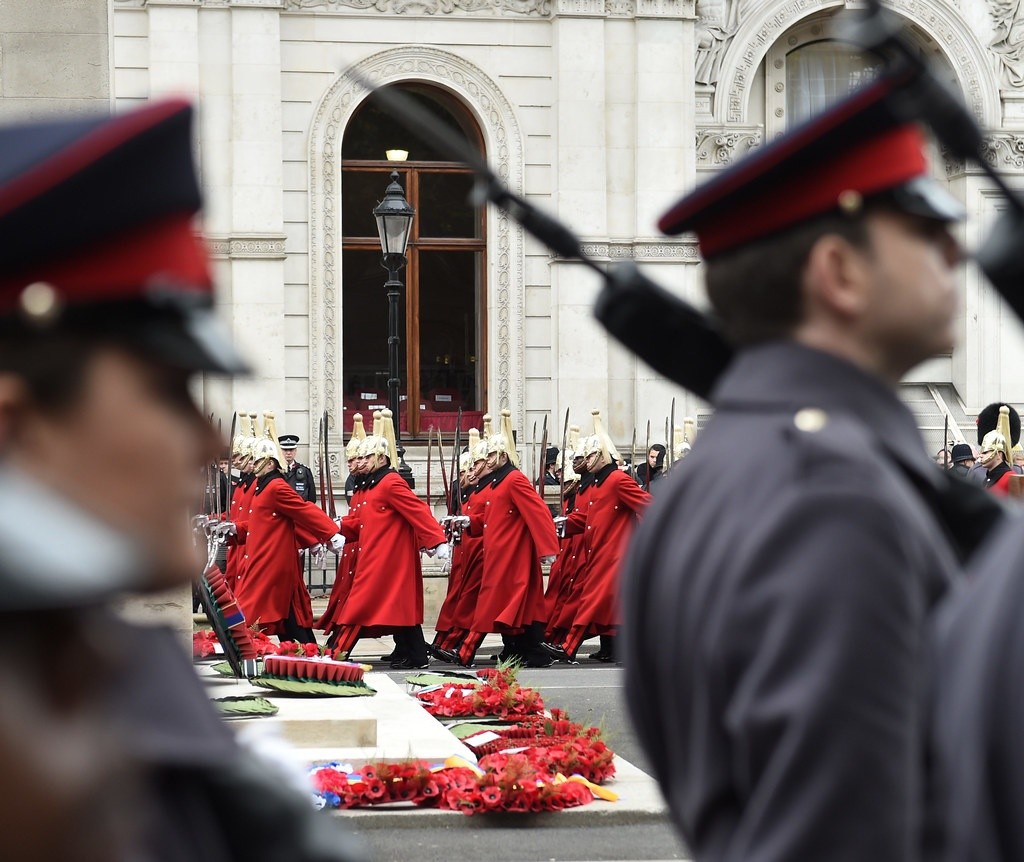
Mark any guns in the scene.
[336,57,1006,575]
[836,0,1024,332]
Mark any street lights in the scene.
[372,168,416,488]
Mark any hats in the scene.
[654,80,970,263]
[278,435,301,450]
[554,408,623,482]
[662,420,695,473]
[949,444,976,463]
[980,405,1013,468]
[0,95,255,382]
[460,408,520,473]
[232,412,285,470]
[343,408,398,471]
[546,446,560,464]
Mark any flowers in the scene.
[192,629,618,812]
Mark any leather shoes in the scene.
[538,640,566,659]
[436,647,460,663]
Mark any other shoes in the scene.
[390,657,429,670]
[587,651,624,666]
[490,654,506,661]
[380,648,400,661]
[426,643,452,663]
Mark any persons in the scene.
[0,97,366,862]
[195,408,692,670]
[616,75,1024,862]
